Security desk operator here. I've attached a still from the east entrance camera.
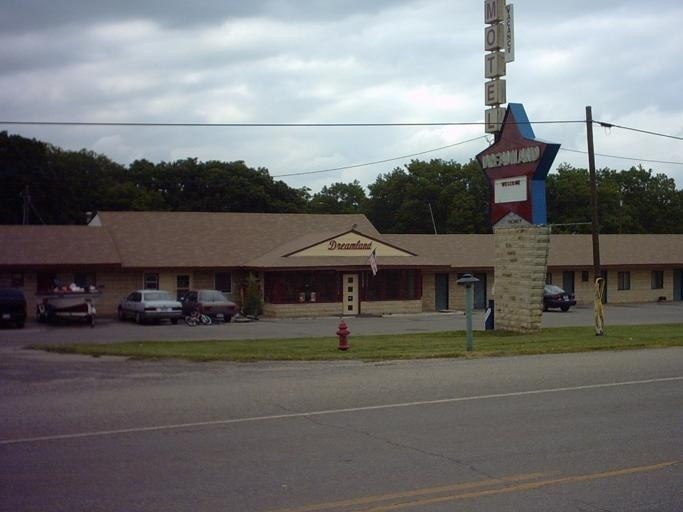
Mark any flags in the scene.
[368,250,378,275]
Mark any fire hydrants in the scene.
[335,319,350,350]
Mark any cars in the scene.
[0,289,27,327]
[543,284,576,312]
[118,289,236,325]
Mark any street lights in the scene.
[456,274,481,351]
